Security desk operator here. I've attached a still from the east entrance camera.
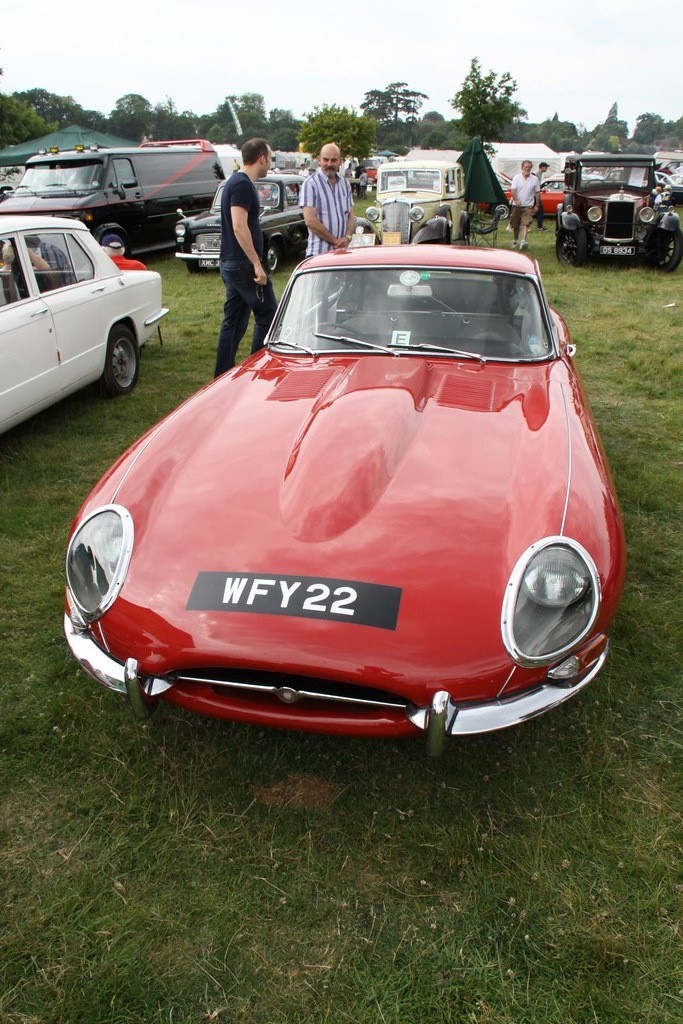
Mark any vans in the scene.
[0,140,244,261]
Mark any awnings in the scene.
[1,123,169,166]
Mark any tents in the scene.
[404,141,613,195]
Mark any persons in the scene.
[297,142,356,260]
[506,160,549,251]
[0,235,77,310]
[214,136,278,378]
[0,234,78,304]
[229,153,404,200]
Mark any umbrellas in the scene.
[377,148,399,156]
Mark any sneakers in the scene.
[526,227,533,232]
[536,227,549,233]
[520,241,527,250]
[506,227,514,232]
[513,241,519,248]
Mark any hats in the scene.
[539,163,549,168]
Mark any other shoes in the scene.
[359,197,367,199]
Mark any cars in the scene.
[1,215,169,442]
[64,244,627,760]
[346,160,473,246]
[245,151,683,219]
[174,172,312,273]
[555,154,682,273]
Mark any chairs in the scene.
[330,314,393,334]
[455,317,521,344]
[472,210,501,248]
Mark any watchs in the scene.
[345,235,352,242]
